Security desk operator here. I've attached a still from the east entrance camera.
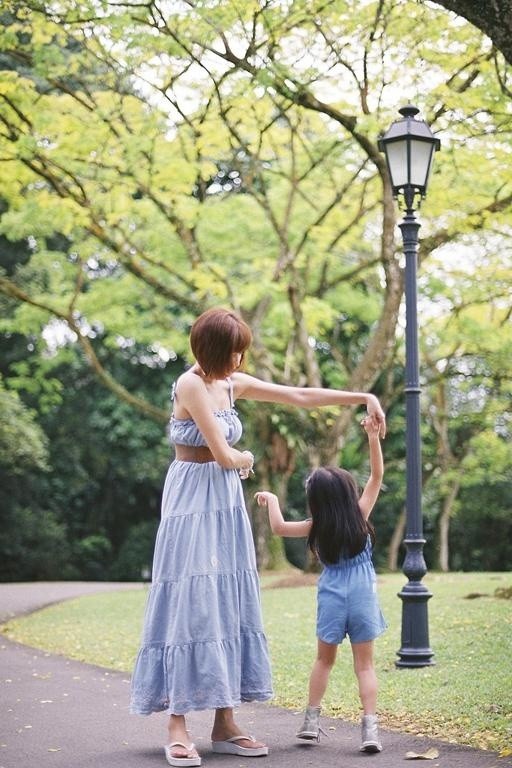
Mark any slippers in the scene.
[211,735,268,756]
[164,742,203,766]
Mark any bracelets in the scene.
[240,448,255,472]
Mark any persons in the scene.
[129,307,388,768]
[252,411,383,758]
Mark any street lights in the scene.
[378,106,441,670]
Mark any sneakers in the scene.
[359,715,383,752]
[295,707,322,739]
